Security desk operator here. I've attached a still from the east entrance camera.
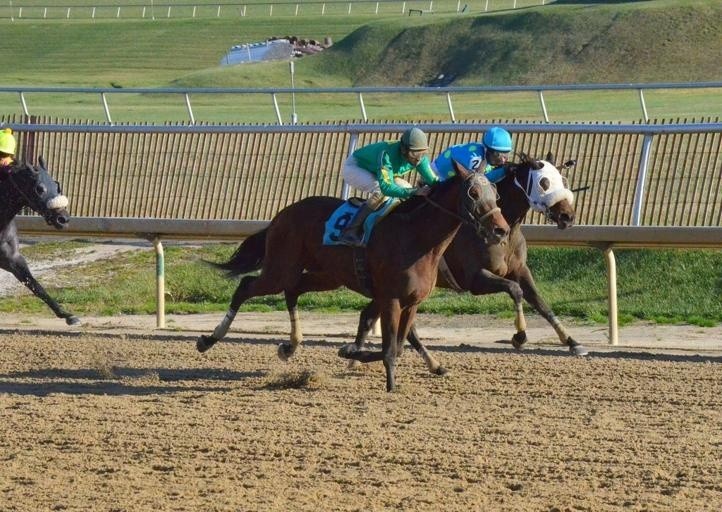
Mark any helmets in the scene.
[0,128,17,156]
[483,127,512,152]
[400,128,429,151]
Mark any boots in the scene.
[342,203,374,246]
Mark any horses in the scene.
[196,160,511,393]
[0,155,79,326]
[344,151,589,376]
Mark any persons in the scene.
[341,126,441,249]
[1,128,20,169]
[416,126,516,195]
